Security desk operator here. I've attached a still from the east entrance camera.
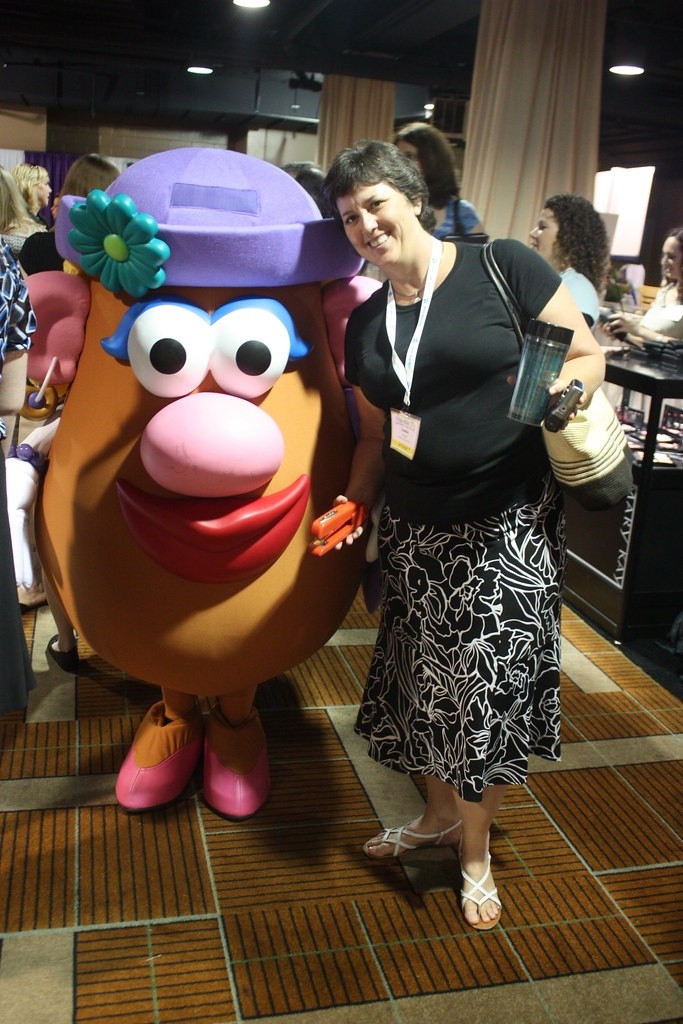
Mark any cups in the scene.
[507,318,575,429]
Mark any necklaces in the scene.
[392,286,424,304]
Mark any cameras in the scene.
[544,379,584,433]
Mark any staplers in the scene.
[307,501,370,558]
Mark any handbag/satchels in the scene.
[479,239,634,510]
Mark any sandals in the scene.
[459,832,502,930]
[363,818,463,860]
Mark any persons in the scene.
[597,226,683,347]
[0,154,122,715]
[279,161,332,220]
[528,192,609,332]
[392,124,484,238]
[321,141,606,930]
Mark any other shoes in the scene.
[47,633,80,672]
